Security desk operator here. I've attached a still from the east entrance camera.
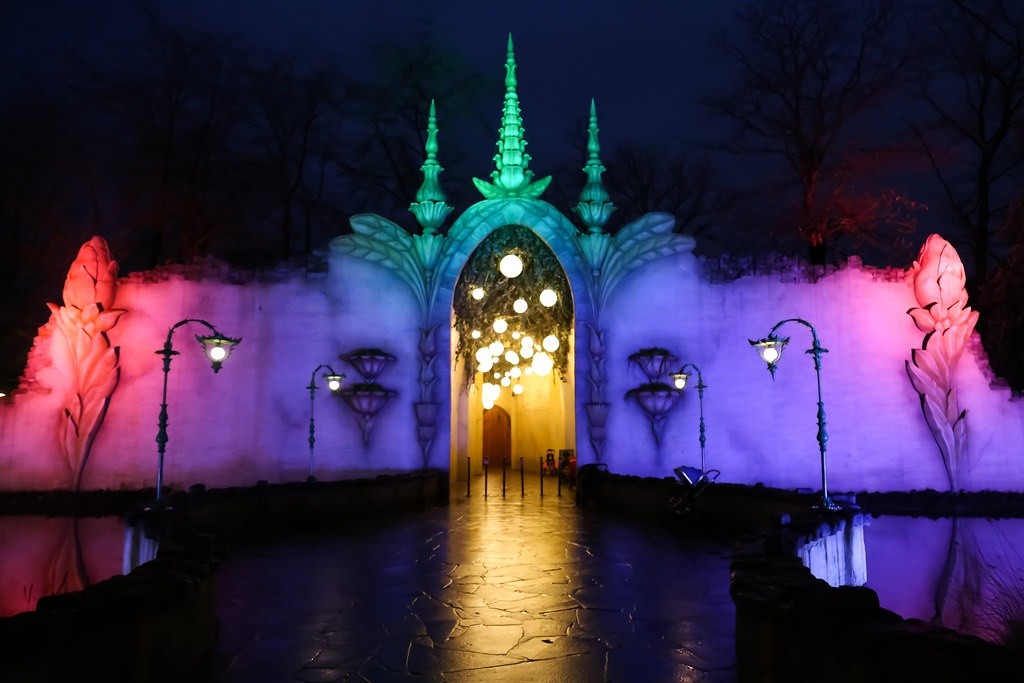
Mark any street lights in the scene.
[309,365,345,478]
[155,318,243,502]
[669,363,708,472]
[747,317,829,501]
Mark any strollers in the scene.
[655,467,722,523]
[574,463,609,511]
